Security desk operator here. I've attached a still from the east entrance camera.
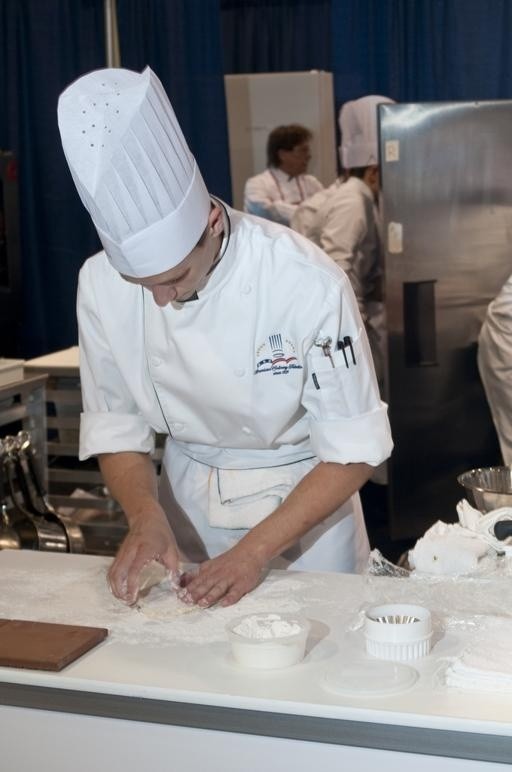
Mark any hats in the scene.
[339,95,397,169]
[56,67,211,279]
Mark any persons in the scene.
[476,274,511,468]
[57,67,397,609]
[242,125,324,230]
[289,94,397,529]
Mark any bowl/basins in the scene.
[229,612,311,665]
[458,465,511,511]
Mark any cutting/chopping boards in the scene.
[1,617,108,675]
